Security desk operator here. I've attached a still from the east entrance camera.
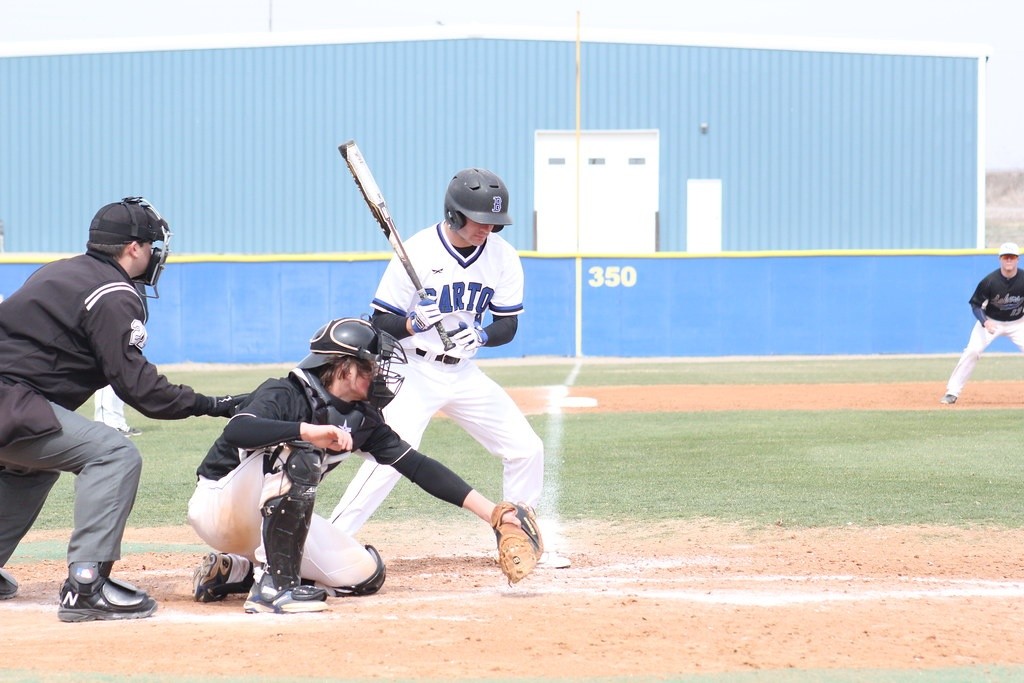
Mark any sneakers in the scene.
[0,572,20,599]
[242,578,332,615]
[192,552,231,602]
[58,581,159,623]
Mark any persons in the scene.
[188,316,541,614]
[0,196,252,622]
[939,241,1024,405]
[323,167,574,569]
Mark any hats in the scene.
[999,242,1020,256]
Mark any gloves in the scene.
[208,392,249,418]
[447,320,488,352]
[410,299,445,333]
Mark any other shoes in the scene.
[941,395,957,404]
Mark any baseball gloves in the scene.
[490,501,545,576]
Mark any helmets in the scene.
[87,201,164,244]
[444,169,514,232]
[296,317,381,370]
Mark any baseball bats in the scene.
[337,136,456,351]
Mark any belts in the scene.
[416,348,461,365]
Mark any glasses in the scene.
[1001,255,1017,260]
